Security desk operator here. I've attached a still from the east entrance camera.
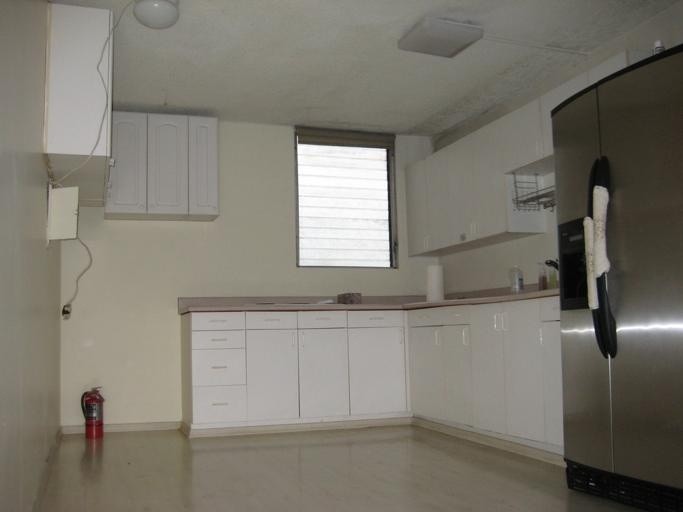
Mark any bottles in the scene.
[510,264,524,292]
[651,40,665,55]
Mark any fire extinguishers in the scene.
[80,387,105,439]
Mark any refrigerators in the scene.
[550,42,683,511]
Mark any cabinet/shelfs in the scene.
[407,295,472,428]
[404,48,642,258]
[42,2,219,222]
[245,312,351,427]
[540,295,563,449]
[178,311,247,430]
[471,299,544,444]
[347,311,408,421]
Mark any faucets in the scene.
[545,259,559,270]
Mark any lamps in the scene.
[396,16,485,59]
[132,0,180,31]
